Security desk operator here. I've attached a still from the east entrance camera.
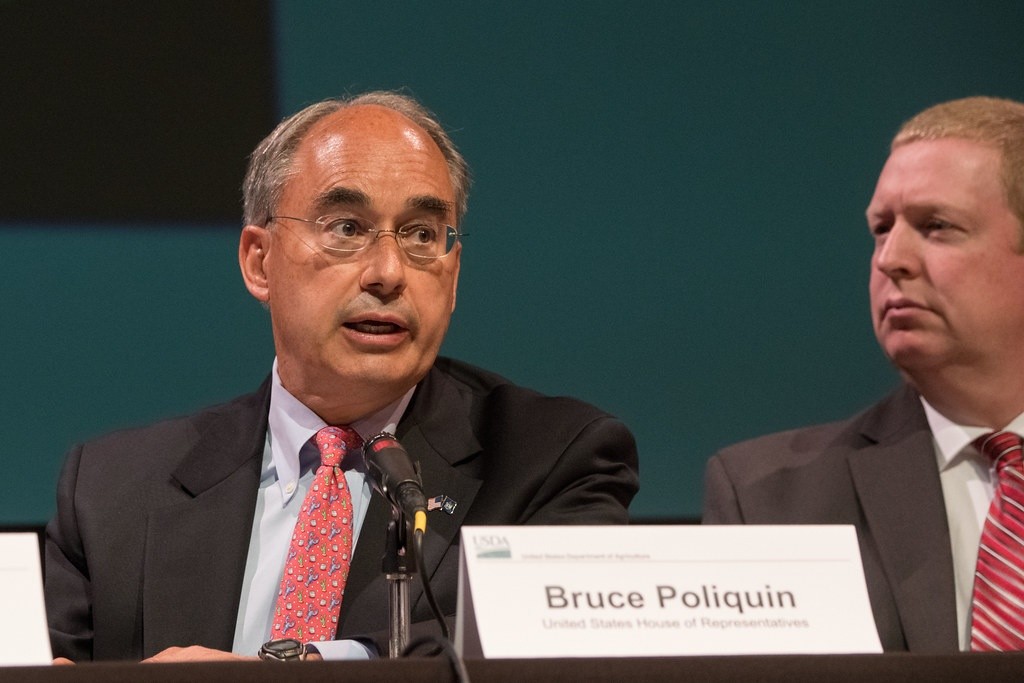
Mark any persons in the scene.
[43,92,640,661]
[711,98,1023,656]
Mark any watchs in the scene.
[257,638,308,663]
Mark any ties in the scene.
[270,426,362,648]
[969,431,1024,651]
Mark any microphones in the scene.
[360,431,427,521]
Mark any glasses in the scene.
[270,216,469,258]
